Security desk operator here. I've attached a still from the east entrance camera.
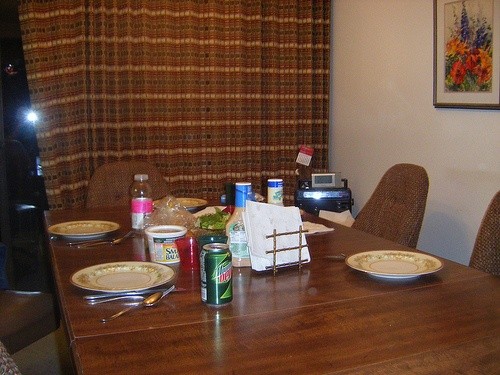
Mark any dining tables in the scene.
[41,199,500,375]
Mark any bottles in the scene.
[225,182,251,267]
[266,178,284,208]
[129,174,153,231]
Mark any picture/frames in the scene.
[432,0,500,110]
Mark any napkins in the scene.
[242,200,311,272]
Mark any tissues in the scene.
[240,198,312,272]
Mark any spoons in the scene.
[80,238,124,249]
[100,291,163,324]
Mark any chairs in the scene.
[0,309,61,375]
[85,160,173,208]
[468,190,500,280]
[349,163,429,249]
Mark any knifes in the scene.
[83,288,178,298]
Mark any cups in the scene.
[196,234,228,255]
[175,237,199,268]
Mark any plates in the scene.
[221,192,265,203]
[345,250,444,278]
[154,198,208,210]
[70,260,177,293]
[47,221,120,239]
[191,206,226,229]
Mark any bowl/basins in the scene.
[145,224,188,263]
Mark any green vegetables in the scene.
[195,206,231,229]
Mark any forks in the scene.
[86,285,176,306]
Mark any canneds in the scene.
[199,243,233,306]
